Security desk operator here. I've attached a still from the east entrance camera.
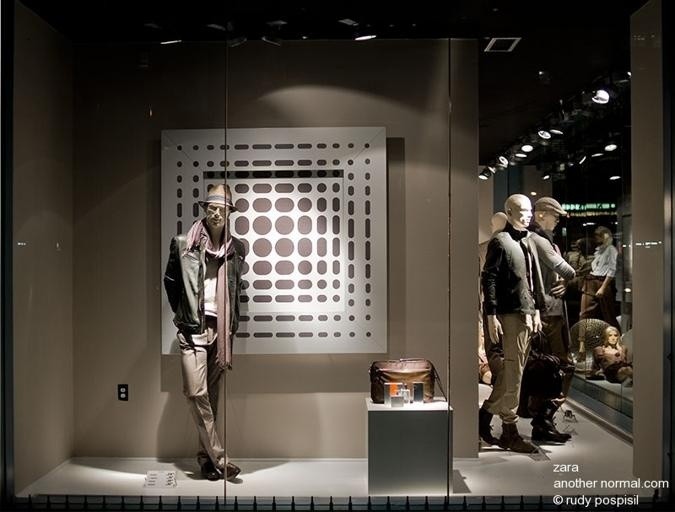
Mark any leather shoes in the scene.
[532,426,571,443]
[201,462,240,480]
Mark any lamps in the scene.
[478,66,630,181]
[157,0,379,47]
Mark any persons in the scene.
[526,197,577,443]
[477,192,545,455]
[478,211,508,385]
[575,225,620,365]
[591,323,633,384]
[163,183,243,480]
[478,311,493,386]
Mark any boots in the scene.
[500,424,538,453]
[479,407,499,444]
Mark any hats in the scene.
[535,197,567,215]
[197,184,237,212]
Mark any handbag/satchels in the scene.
[523,353,562,399]
[370,357,435,403]
